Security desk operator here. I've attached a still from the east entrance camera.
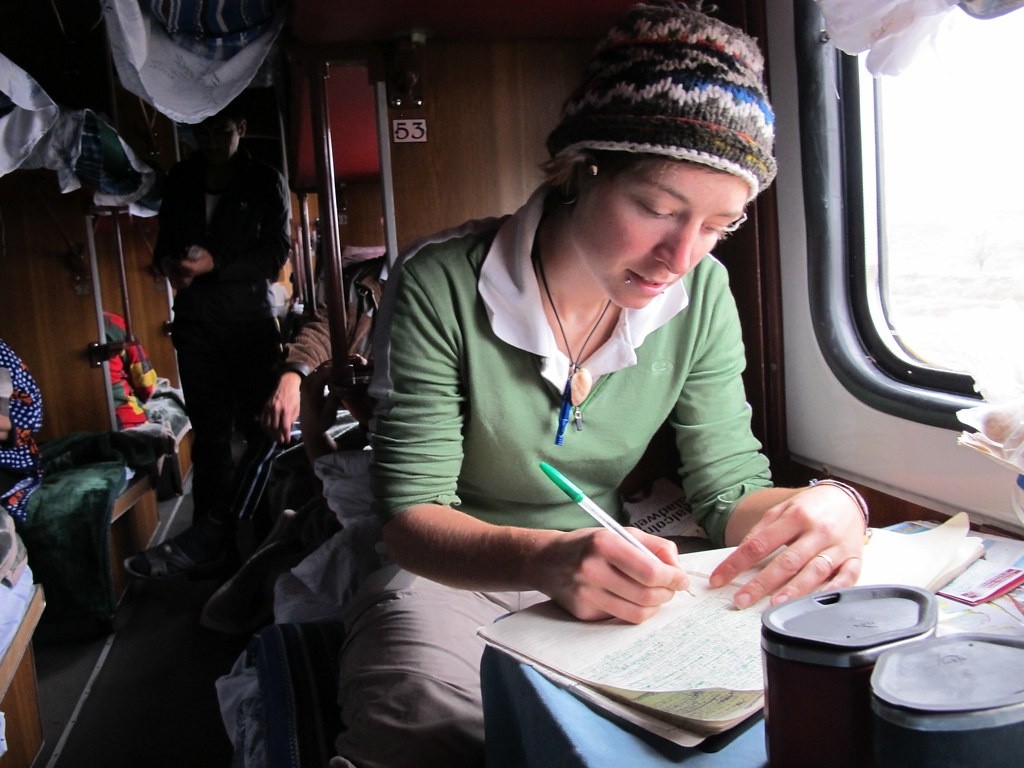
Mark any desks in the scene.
[480,522,1024,768]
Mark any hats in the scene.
[546,6,778,206]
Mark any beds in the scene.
[1,417,192,768]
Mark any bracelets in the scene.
[806,476,871,543]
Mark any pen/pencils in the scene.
[537,458,701,601]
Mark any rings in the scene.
[817,551,837,575]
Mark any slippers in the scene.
[120,540,208,575]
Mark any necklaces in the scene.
[533,230,614,444]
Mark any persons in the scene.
[103,310,183,501]
[153,115,290,531]
[123,252,388,589]
[0,339,42,548]
[321,6,871,768]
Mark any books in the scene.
[478,512,984,752]
